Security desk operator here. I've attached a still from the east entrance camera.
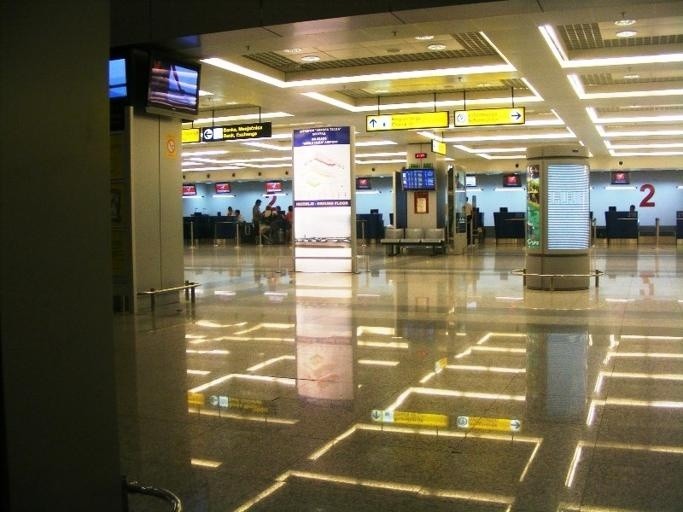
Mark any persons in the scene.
[253,199,293,235]
[226,206,244,222]
[466,196,473,246]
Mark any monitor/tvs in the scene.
[503,175,521,187]
[215,182,231,194]
[401,169,435,190]
[145,50,201,115]
[182,184,196,196]
[612,172,629,184]
[109,55,133,105]
[356,177,370,189]
[457,175,477,187]
[265,181,282,193]
